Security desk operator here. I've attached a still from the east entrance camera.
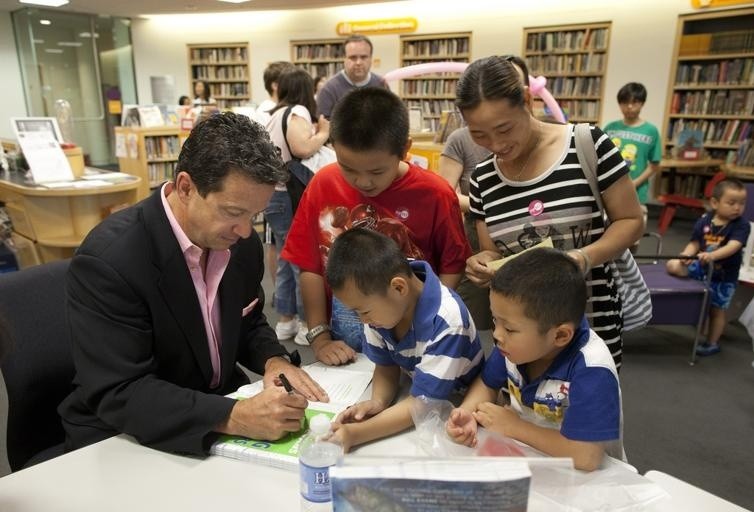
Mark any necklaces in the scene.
[199,251,209,256]
[710,215,730,241]
[501,121,545,182]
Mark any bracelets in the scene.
[305,322,333,344]
[571,247,591,278]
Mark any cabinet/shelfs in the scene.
[2,139,145,273]
[290,39,358,80]
[521,21,613,128]
[653,3,753,213]
[185,41,253,107]
[115,122,186,202]
[399,31,474,135]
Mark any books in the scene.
[190,45,253,107]
[523,28,611,123]
[201,363,374,471]
[328,457,533,512]
[291,42,353,79]
[145,134,181,181]
[401,38,472,134]
[657,32,754,200]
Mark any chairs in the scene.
[658,168,725,228]
[604,233,711,370]
[2,258,122,468]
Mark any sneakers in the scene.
[294,325,311,346]
[275,319,302,341]
[696,343,720,355]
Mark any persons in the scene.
[59,112,333,460]
[665,175,752,356]
[445,245,628,474]
[324,227,490,454]
[278,87,475,365]
[177,53,754,350]
[313,34,391,152]
[453,54,647,377]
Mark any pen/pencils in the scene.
[279,372,308,420]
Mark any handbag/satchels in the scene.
[285,145,341,215]
[609,249,652,332]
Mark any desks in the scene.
[649,154,718,223]
[1,354,753,511]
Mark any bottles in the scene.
[296,412,347,508]
